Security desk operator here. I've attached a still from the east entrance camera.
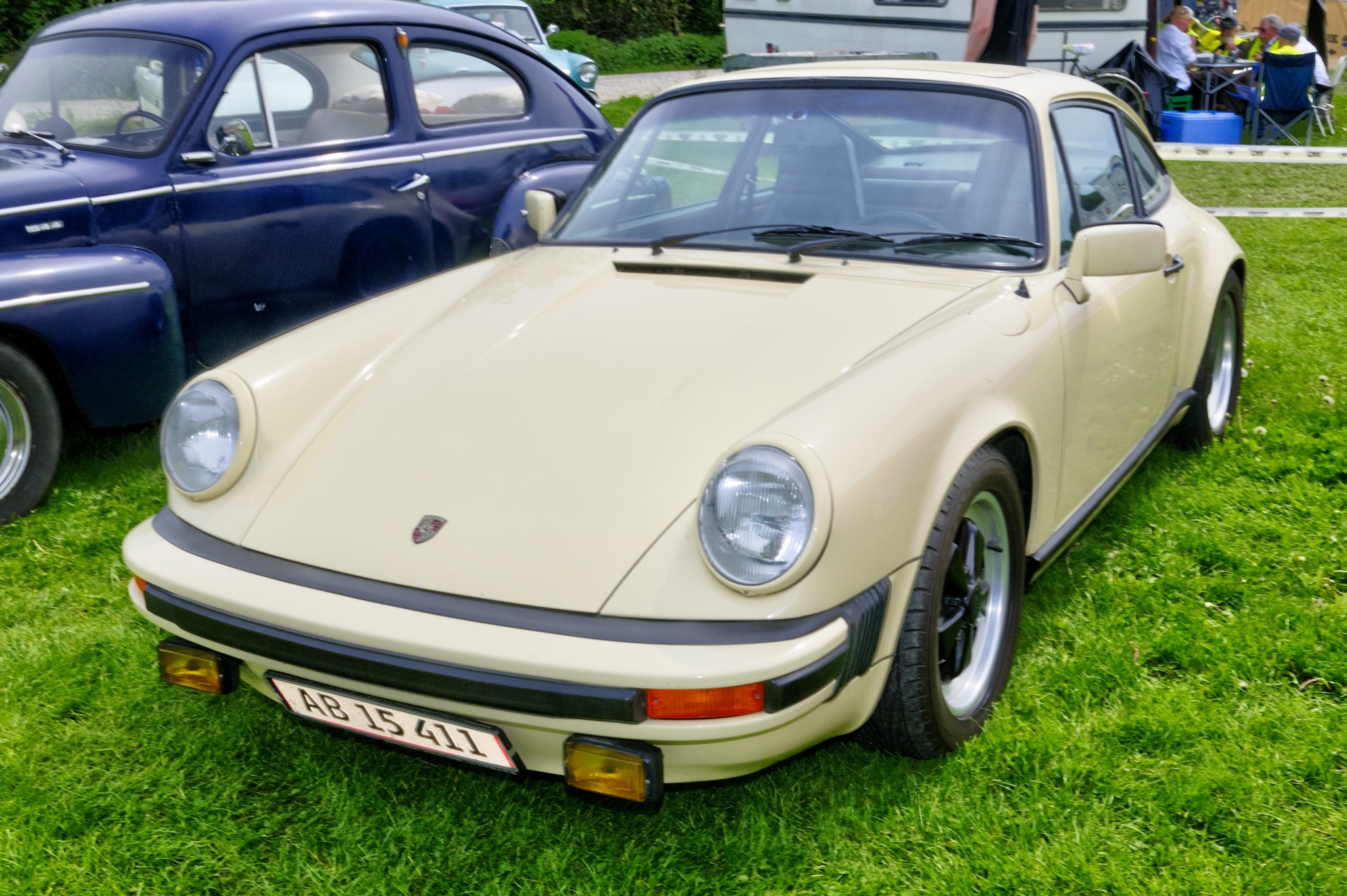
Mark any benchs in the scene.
[297,109,511,142]
[861,166,974,222]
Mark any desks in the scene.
[1187,58,1264,144]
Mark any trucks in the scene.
[715,0,1158,102]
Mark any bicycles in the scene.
[1012,43,1152,131]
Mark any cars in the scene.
[0,1,675,533]
[120,45,1249,820]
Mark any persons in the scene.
[1157,6,1331,136]
[964,0,1040,66]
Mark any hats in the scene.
[1218,17,1238,29]
[1279,26,1300,40]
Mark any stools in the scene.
[1168,95,1192,110]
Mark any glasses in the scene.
[1259,28,1272,33]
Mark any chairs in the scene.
[757,133,859,227]
[956,142,1036,242]
[1235,51,1347,147]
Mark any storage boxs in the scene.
[1157,110,1244,145]
